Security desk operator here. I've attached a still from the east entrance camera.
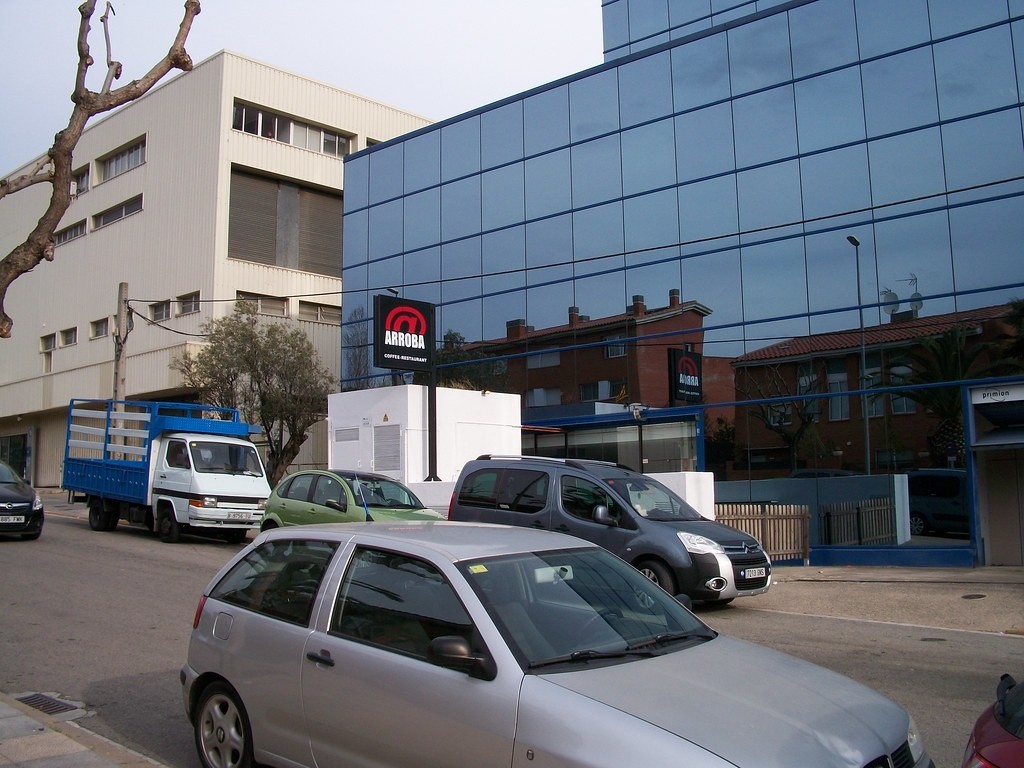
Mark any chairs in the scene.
[293,487,307,500]
[469,568,557,675]
[340,571,429,662]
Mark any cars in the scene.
[259,470,448,532]
[179,519,935,768]
[961,673,1024,768]
[0,460,45,541]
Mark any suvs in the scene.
[902,468,971,536]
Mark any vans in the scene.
[447,455,772,612]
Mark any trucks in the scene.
[60,398,272,545]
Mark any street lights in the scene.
[847,235,870,474]
